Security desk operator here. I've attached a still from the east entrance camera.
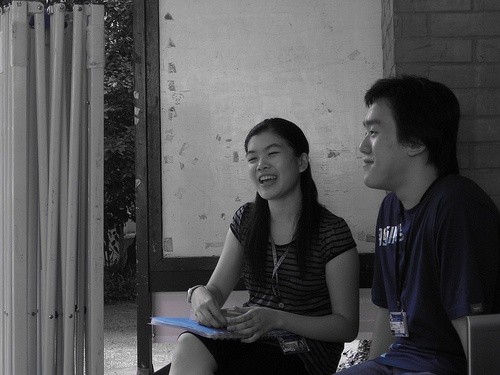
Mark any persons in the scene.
[168,117,360,375]
[334,74,500,375]
[297,341,307,353]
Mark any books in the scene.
[148,317,293,340]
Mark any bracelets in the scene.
[187,285,204,304]
